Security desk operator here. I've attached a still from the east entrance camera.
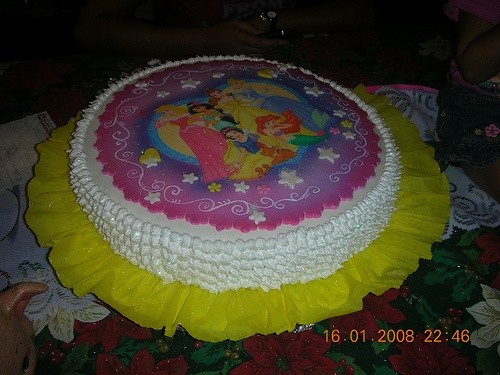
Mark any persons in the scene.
[71,0,363,58]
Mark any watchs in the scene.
[441,1,499,79]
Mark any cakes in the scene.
[65,54,403,296]
